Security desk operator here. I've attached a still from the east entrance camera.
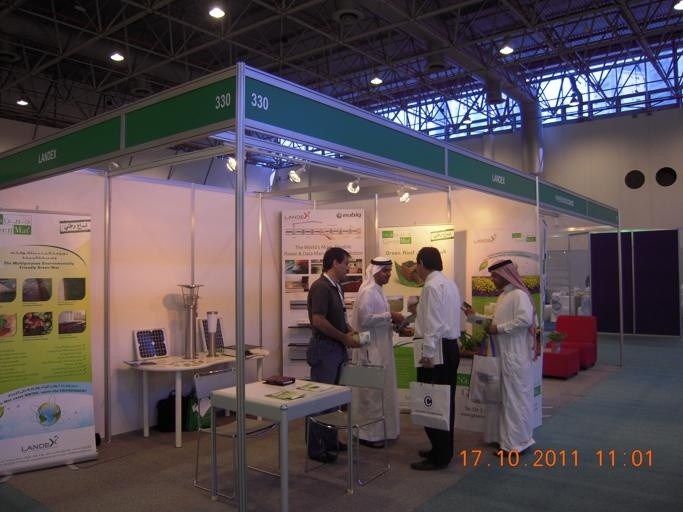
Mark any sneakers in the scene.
[492,447,513,459]
[358,438,385,448]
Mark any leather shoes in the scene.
[331,440,348,453]
[318,452,338,464]
[419,448,431,456]
[410,461,444,470]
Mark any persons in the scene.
[412,247,462,471]
[351,257,406,449]
[460,258,538,458]
[305,246,357,462]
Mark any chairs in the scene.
[193,367,282,499]
[305,361,390,486]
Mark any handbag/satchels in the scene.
[468,329,503,405]
[410,362,450,431]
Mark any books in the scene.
[345,332,370,348]
[266,391,304,400]
[460,300,471,311]
[411,337,444,368]
[462,312,493,328]
[263,375,296,386]
[297,384,332,393]
[399,310,413,330]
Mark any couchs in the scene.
[554,314,598,368]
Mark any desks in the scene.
[122,349,270,449]
[210,378,354,512]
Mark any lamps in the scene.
[346,175,363,193]
[397,186,410,204]
[287,163,308,183]
